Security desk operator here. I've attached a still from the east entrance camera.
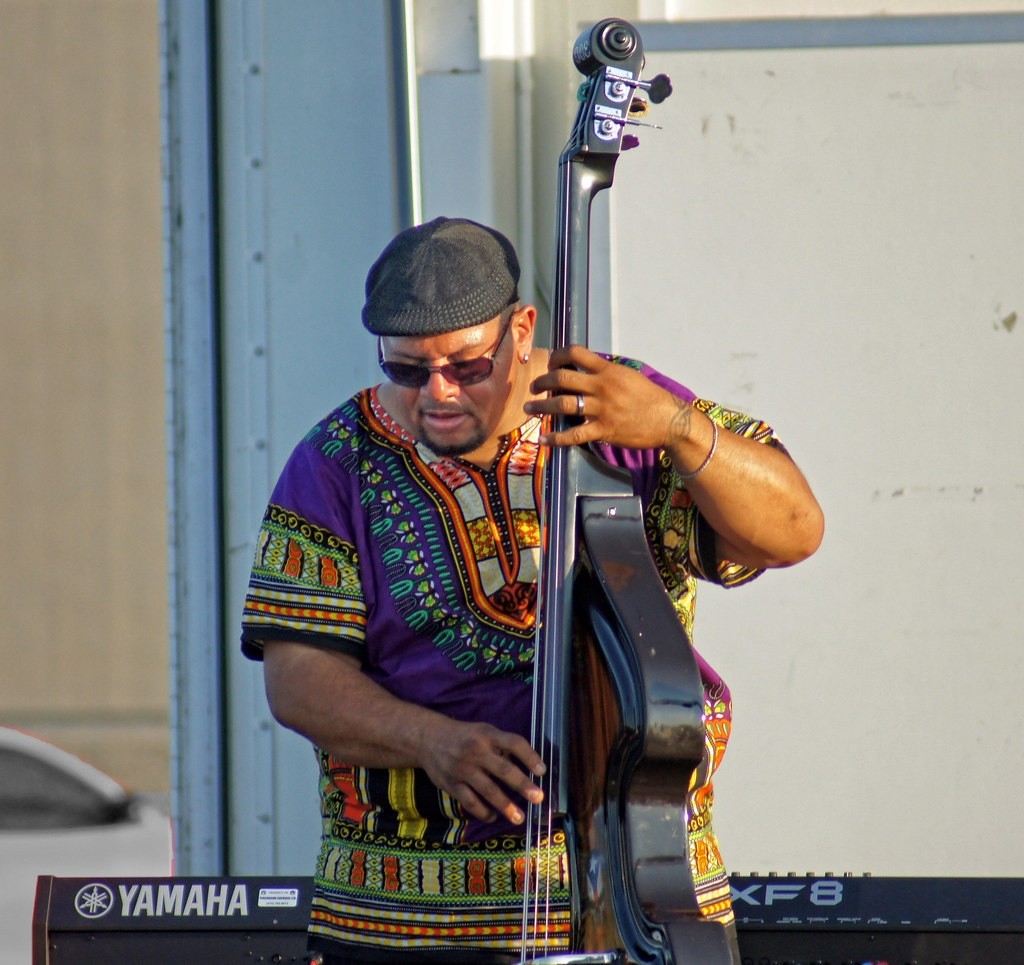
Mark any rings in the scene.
[576,393,585,416]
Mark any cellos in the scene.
[509,14,751,965]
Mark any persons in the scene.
[241,213,824,964]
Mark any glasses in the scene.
[377,311,514,387]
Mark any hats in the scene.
[360,214,521,337]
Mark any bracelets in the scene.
[675,412,720,479]
